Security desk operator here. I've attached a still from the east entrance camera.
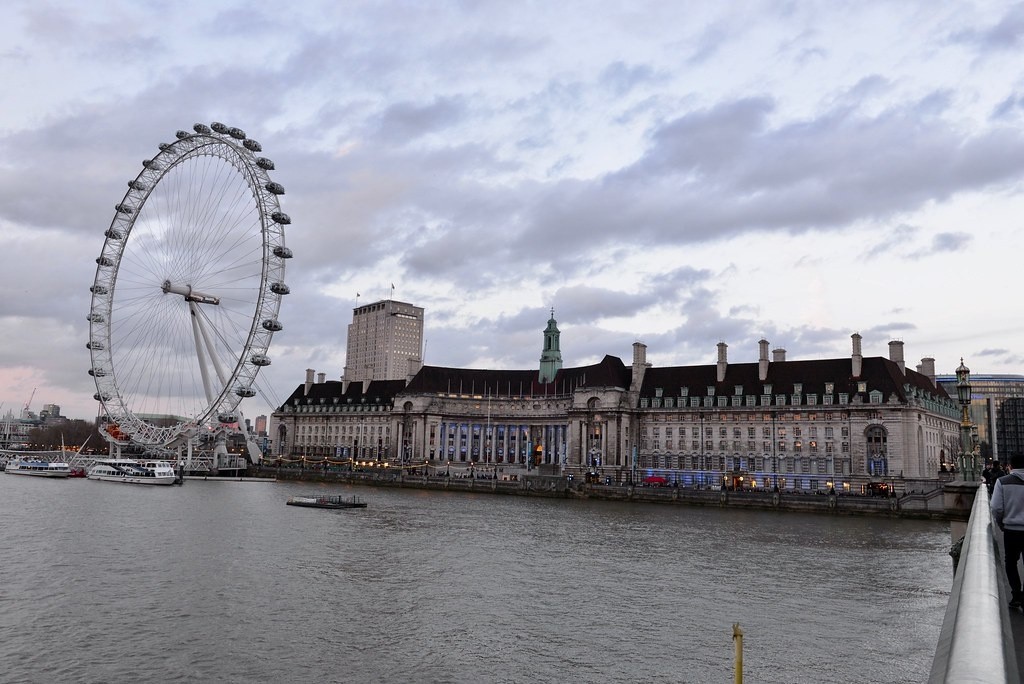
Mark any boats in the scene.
[86,459,177,485]
[69,465,89,477]
[5,460,70,478]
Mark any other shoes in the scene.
[1009,595,1022,607]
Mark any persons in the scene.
[990,454,1024,610]
[982,459,1012,497]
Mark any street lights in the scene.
[955,356,973,482]
